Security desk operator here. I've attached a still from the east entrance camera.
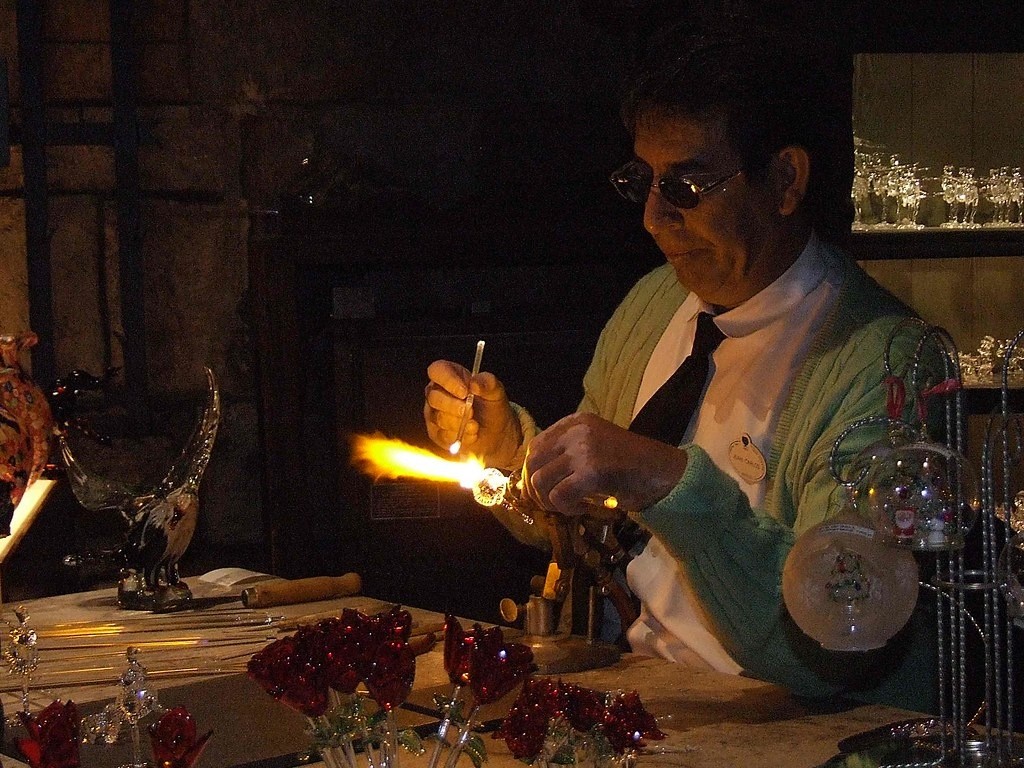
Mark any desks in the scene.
[0,569,1024,768]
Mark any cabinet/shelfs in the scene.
[850,0,1024,537]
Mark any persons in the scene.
[424,23,964,717]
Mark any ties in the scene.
[572,312,725,641]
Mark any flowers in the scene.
[1,603,669,768]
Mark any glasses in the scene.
[609,153,761,211]
